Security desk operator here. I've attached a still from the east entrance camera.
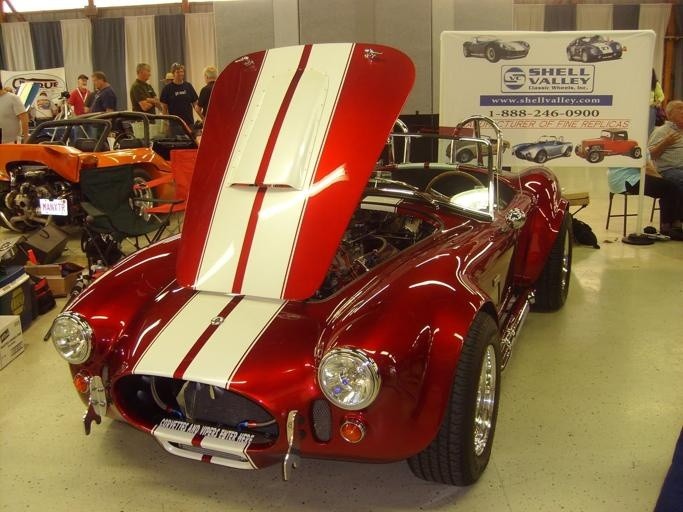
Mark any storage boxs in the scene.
[0,262,84,372]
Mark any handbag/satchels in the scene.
[572,218,598,246]
[26,272,56,317]
[22,222,69,265]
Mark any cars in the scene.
[575,128,642,164]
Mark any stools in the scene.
[605,191,662,237]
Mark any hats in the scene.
[78,74,88,80]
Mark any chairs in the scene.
[79,149,200,279]
[73,136,152,152]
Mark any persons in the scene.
[128,61,161,125]
[197,64,218,118]
[650,68,664,116]
[89,72,117,112]
[646,98,682,240]
[66,73,92,115]
[608,165,667,237]
[161,64,202,135]
[0,81,29,144]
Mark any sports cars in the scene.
[462,36,530,63]
[445,135,510,162]
[566,35,623,64]
[1,109,201,233]
[51,43,574,487]
[511,135,573,164]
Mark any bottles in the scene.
[95,259,105,279]
[27,249,37,264]
